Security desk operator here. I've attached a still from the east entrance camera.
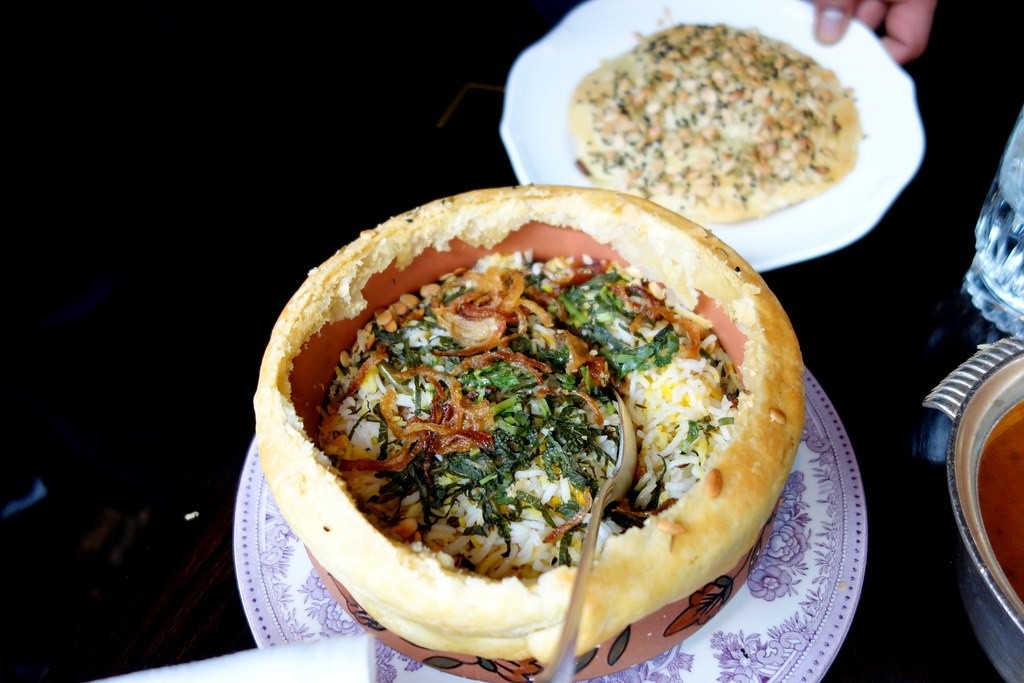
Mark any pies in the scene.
[251,184,807,659]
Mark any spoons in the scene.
[525,387,640,683]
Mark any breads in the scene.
[568,23,863,222]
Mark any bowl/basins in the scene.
[911,335,1024,683]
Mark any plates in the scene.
[498,0,927,273]
[231,365,868,683]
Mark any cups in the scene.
[964,102,1024,335]
